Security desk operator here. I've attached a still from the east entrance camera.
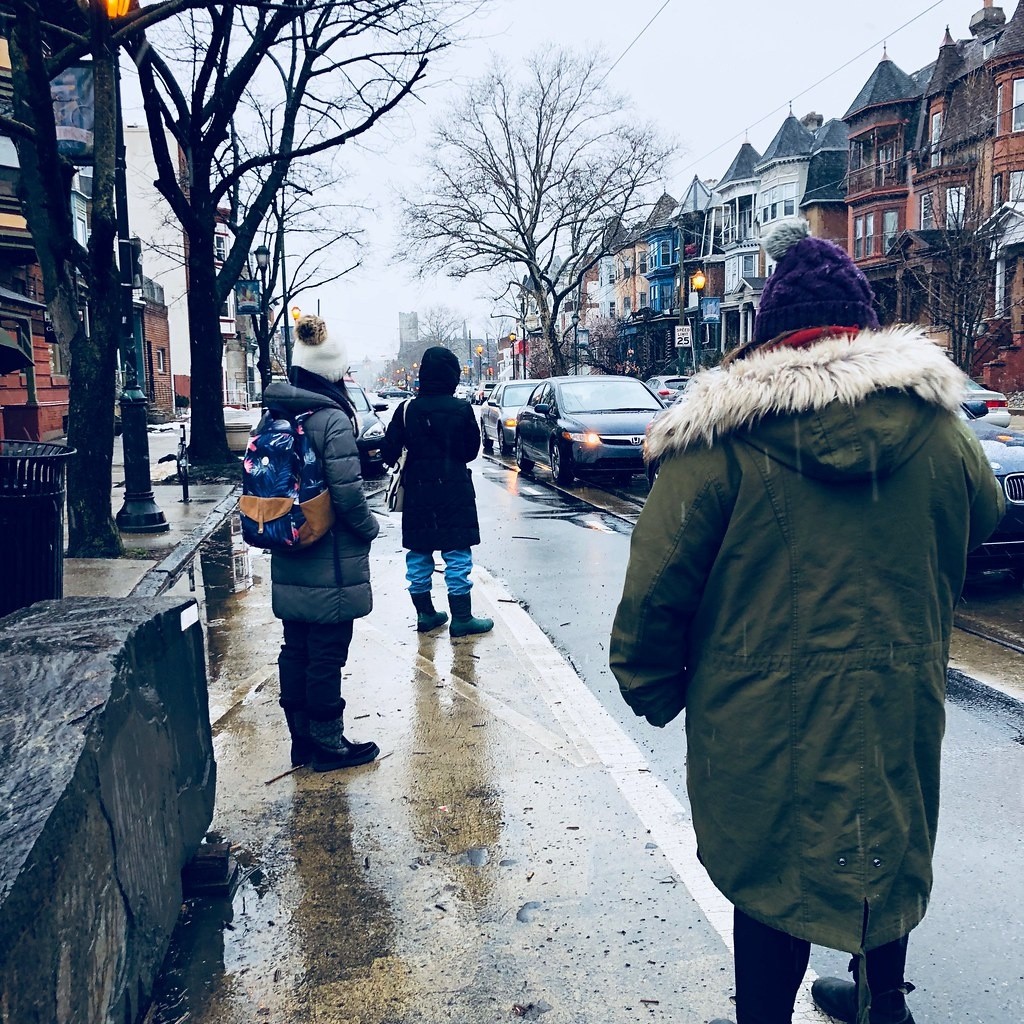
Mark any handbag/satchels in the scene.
[383,397,419,512]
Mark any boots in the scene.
[410,591,448,632]
[279,698,324,765]
[809,958,915,1024]
[303,697,380,773]
[447,591,494,637]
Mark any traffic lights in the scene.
[101,0,139,25]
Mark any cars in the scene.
[958,402,1024,576]
[516,375,669,486]
[956,378,1011,428]
[344,375,391,475]
[454,386,480,404]
[377,386,413,399]
[480,379,546,456]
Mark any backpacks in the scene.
[235,405,343,551]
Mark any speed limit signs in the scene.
[674,325,691,347]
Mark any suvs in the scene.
[472,383,497,405]
[644,375,690,408]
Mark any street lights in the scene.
[572,313,581,375]
[477,345,483,381]
[692,268,706,373]
[254,245,272,416]
[510,333,516,379]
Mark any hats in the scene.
[290,314,351,383]
[751,215,880,344]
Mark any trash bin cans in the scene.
[0,438,77,620]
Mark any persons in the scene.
[380,346,493,636]
[618,359,655,380]
[265,315,380,770]
[609,221,1006,1024]
